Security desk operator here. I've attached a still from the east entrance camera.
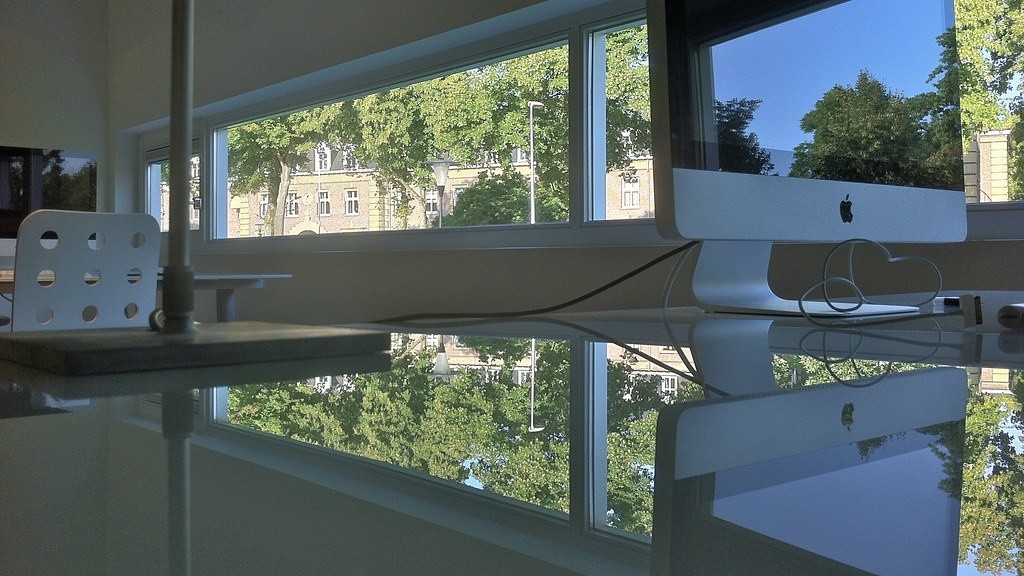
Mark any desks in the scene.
[0,270,291,322]
[0,291,1024,576]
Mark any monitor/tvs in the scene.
[650,310,970,576]
[645,0,967,315]
[0,145,105,270]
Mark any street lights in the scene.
[529,338,546,434]
[527,101,548,223]
[423,333,461,378]
[423,154,461,228]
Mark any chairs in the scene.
[10,209,160,332]
